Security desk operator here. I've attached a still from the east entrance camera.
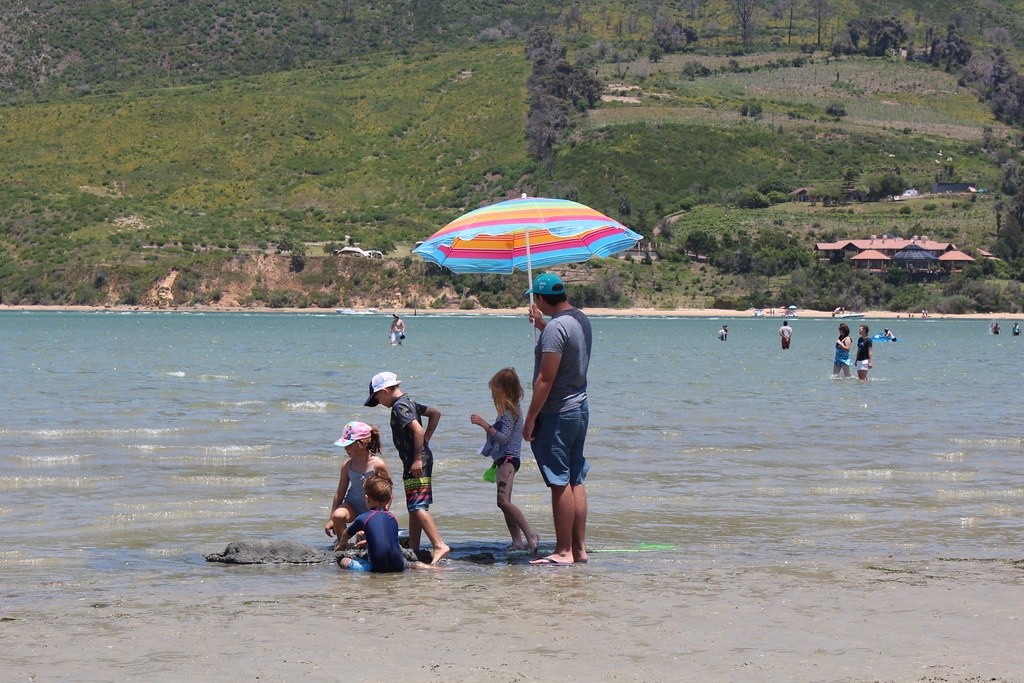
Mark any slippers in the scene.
[530,557,572,566]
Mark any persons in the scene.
[750,307,928,382]
[326,273,599,573]
[991,322,1001,336]
[720,324,728,341]
[1010,322,1023,337]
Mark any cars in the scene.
[332,246,384,267]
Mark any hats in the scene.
[334,421,373,447]
[525,274,565,295]
[364,372,401,407]
[393,313,399,318]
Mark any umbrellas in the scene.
[410,192,644,395]
[789,305,797,316]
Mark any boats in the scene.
[841,314,866,319]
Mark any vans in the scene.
[901,189,918,196]
